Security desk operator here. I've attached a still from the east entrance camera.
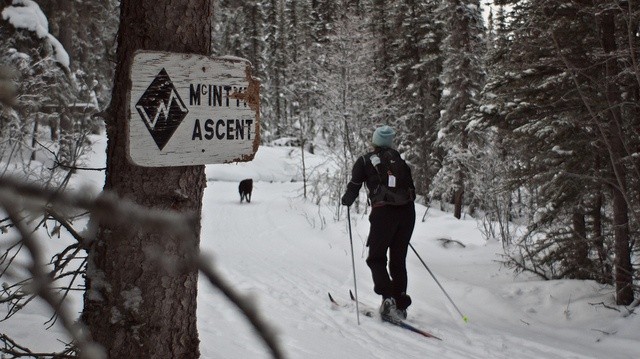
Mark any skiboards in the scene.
[328,288,442,342]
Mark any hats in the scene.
[371,125,396,147]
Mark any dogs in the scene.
[239,178,253,203]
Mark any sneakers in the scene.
[379,294,396,316]
[394,305,408,319]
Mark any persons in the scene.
[342,127,415,318]
